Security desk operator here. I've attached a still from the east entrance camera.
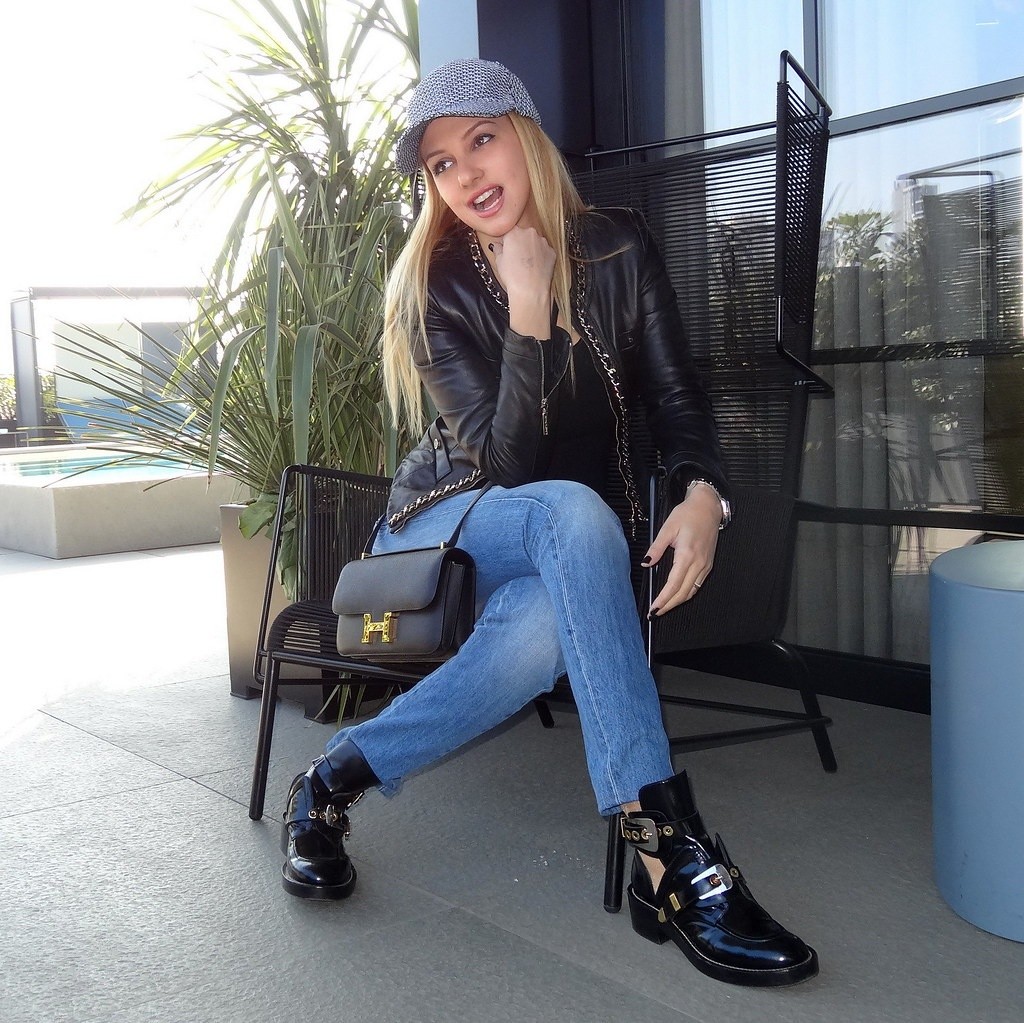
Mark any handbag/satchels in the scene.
[332,543,477,664]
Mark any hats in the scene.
[394,58,542,176]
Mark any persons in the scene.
[280,57,817,987]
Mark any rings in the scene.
[693,582,702,590]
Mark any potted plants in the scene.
[0,0,419,724]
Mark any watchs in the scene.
[684,477,732,530]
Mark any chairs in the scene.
[249,50,837,914]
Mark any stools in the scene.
[929,541,1024,943]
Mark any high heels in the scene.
[627,768,821,989]
[280,740,381,901]
